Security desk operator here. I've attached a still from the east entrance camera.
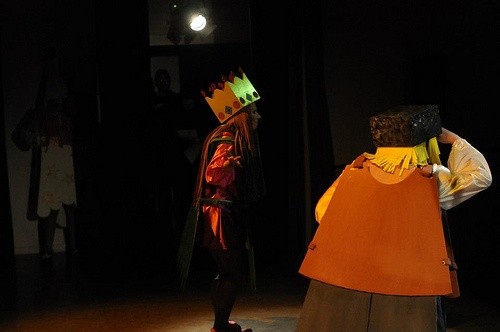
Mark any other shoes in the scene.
[242,329,252,332]
[211,323,241,332]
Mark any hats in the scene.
[201,67,261,124]
[364,105,443,176]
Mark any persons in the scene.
[176,67,261,332]
[11,80,79,274]
[296,104,492,332]
[154,69,175,95]
